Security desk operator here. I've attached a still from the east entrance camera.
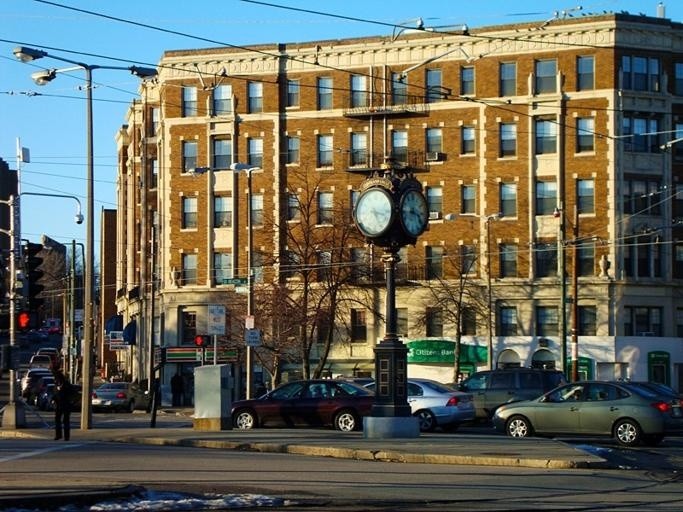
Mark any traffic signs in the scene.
[222,278,247,284]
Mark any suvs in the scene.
[455,369,569,424]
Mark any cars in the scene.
[92,378,149,412]
[231,379,378,432]
[350,377,476,432]
[494,383,681,445]
[5,319,80,414]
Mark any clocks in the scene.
[353,185,430,238]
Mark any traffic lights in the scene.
[17,309,32,330]
[194,334,209,348]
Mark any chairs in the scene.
[596,391,606,400]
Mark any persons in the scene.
[50,373,80,440]
[75,352,194,410]
[573,390,583,401]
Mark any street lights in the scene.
[446,210,508,372]
[10,40,160,430]
[188,160,255,400]
[554,200,570,385]
